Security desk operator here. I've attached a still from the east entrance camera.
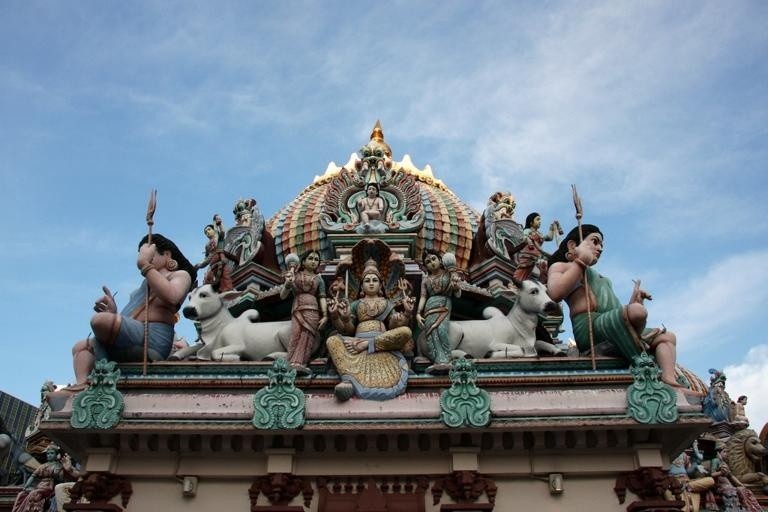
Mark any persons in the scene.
[279,249,327,368]
[71,234,197,385]
[326,257,413,401]
[710,443,744,512]
[194,214,231,293]
[416,248,461,365]
[12,442,63,512]
[668,442,714,493]
[507,212,553,288]
[356,183,384,223]
[547,224,683,388]
[735,396,747,421]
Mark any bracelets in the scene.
[140,263,154,276]
[342,317,349,322]
[416,311,421,314]
[284,282,291,289]
[331,314,338,320]
[550,226,553,231]
[576,258,588,270]
[321,311,327,317]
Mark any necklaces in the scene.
[366,197,376,209]
